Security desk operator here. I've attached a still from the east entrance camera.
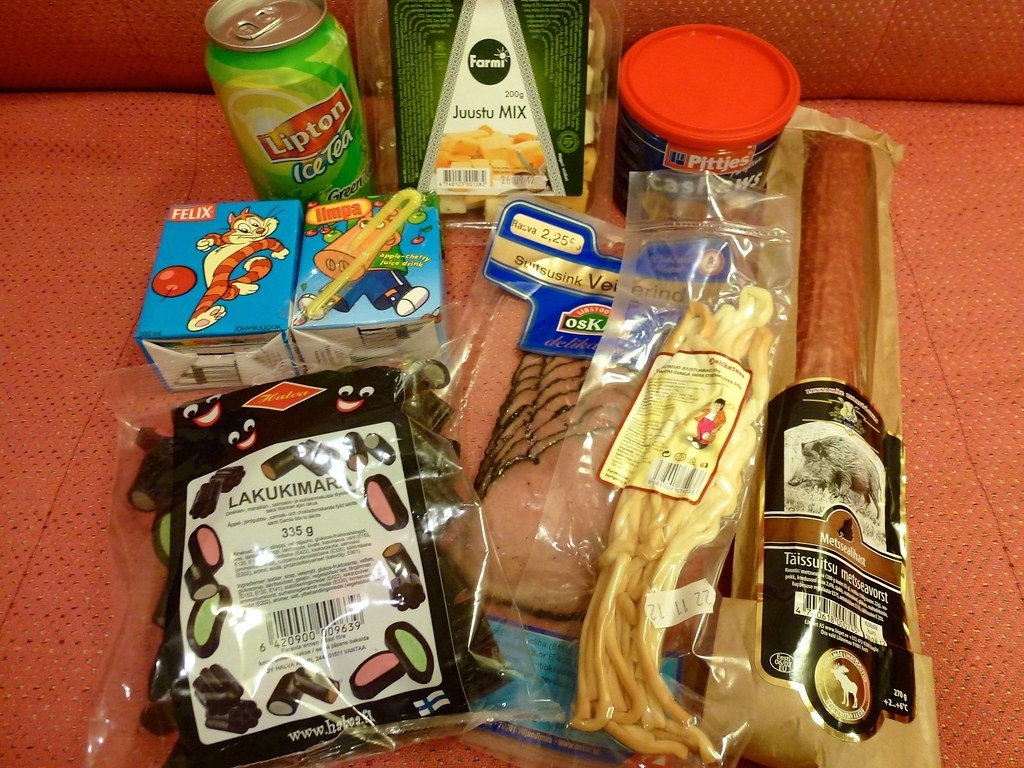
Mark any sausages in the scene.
[793,130,878,400]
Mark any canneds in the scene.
[203,0,377,210]
